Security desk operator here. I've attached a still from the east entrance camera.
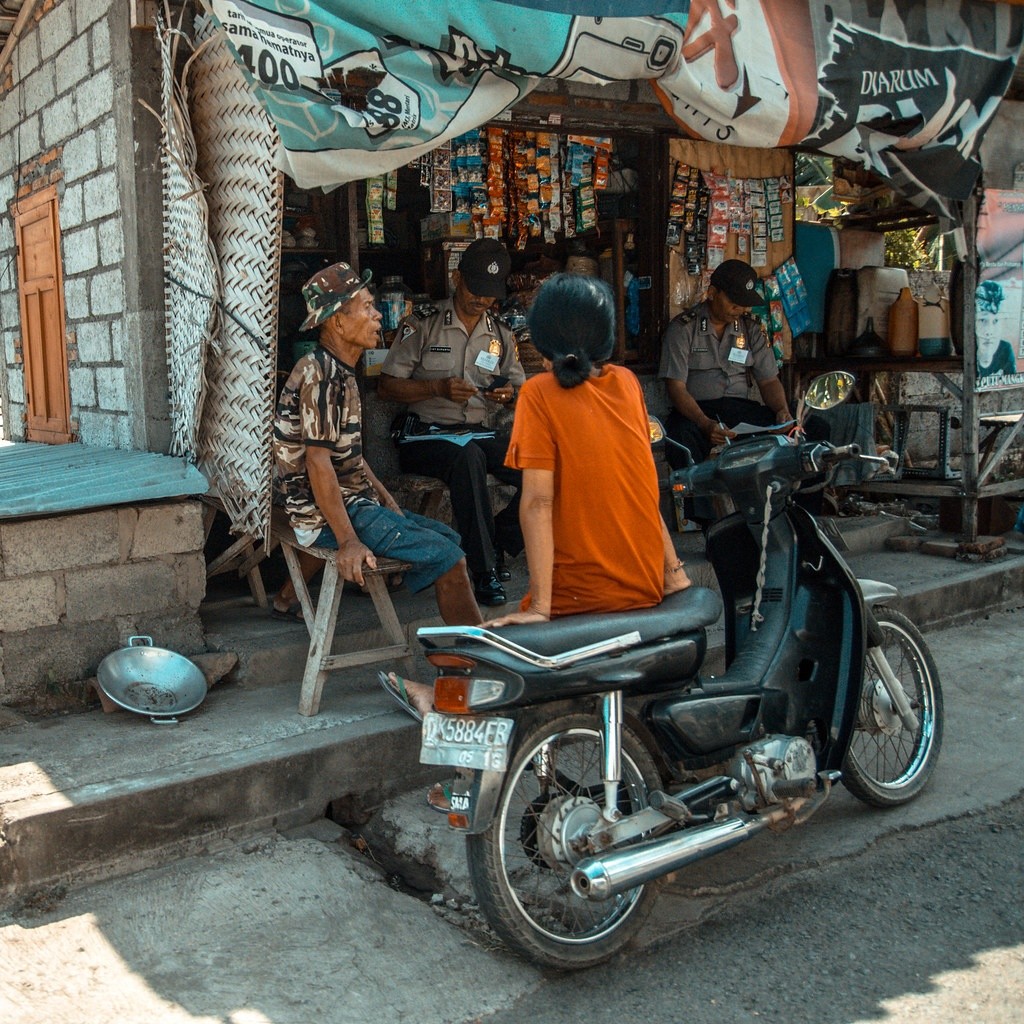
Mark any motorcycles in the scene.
[410,369,944,973]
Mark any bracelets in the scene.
[506,385,517,404]
[665,558,685,573]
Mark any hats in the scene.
[462,237,511,299]
[298,262,372,331]
[710,259,765,307]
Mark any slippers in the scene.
[386,573,408,593]
[272,603,317,623]
[377,669,422,725]
[427,783,453,813]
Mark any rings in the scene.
[498,398,499,401]
[501,394,505,399]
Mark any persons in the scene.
[378,269,691,814]
[273,262,485,643]
[271,551,324,624]
[378,238,526,607]
[657,259,830,531]
[974,281,1017,387]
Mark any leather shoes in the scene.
[495,545,512,581]
[468,568,506,607]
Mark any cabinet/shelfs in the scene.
[798,195,1024,542]
[282,177,360,346]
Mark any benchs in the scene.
[382,443,733,586]
[196,492,420,717]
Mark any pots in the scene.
[97,635,208,725]
